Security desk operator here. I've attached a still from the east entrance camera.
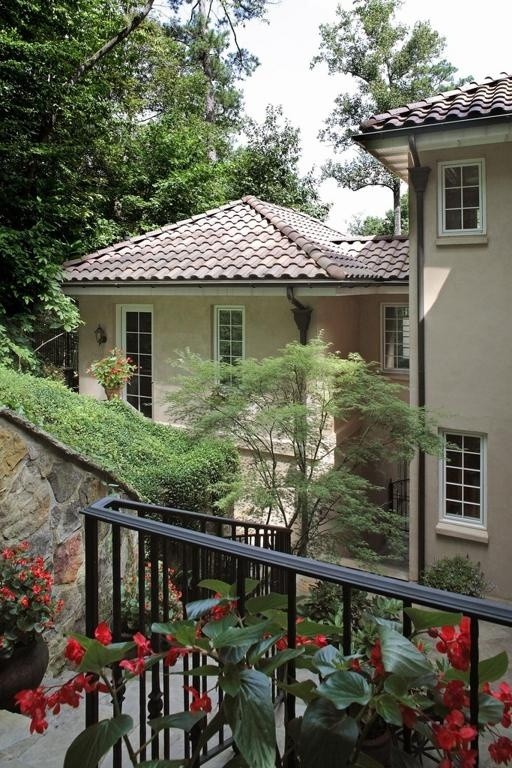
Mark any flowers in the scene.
[87,344,138,388]
[0,540,65,662]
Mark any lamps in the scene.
[94,324,107,346]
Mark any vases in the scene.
[103,386,122,403]
[0,633,50,714]
